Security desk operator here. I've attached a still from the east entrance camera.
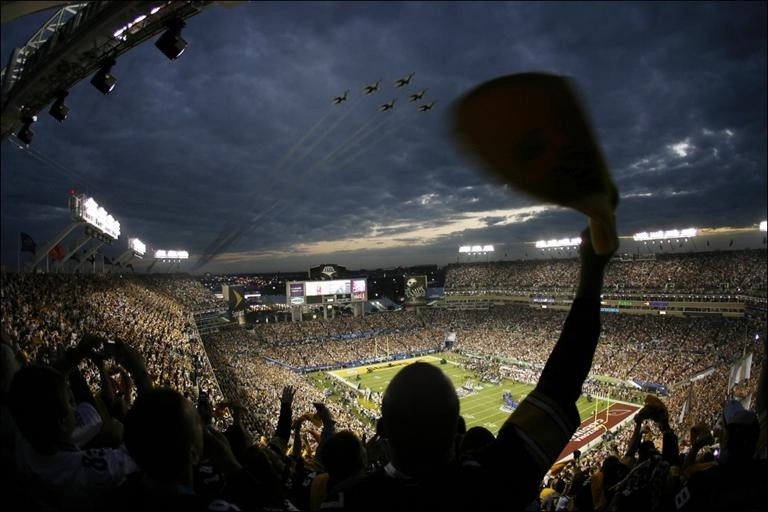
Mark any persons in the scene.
[2,217,767,511]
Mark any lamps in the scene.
[17,19,189,145]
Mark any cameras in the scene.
[638,403,663,422]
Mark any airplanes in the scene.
[332,70,438,114]
